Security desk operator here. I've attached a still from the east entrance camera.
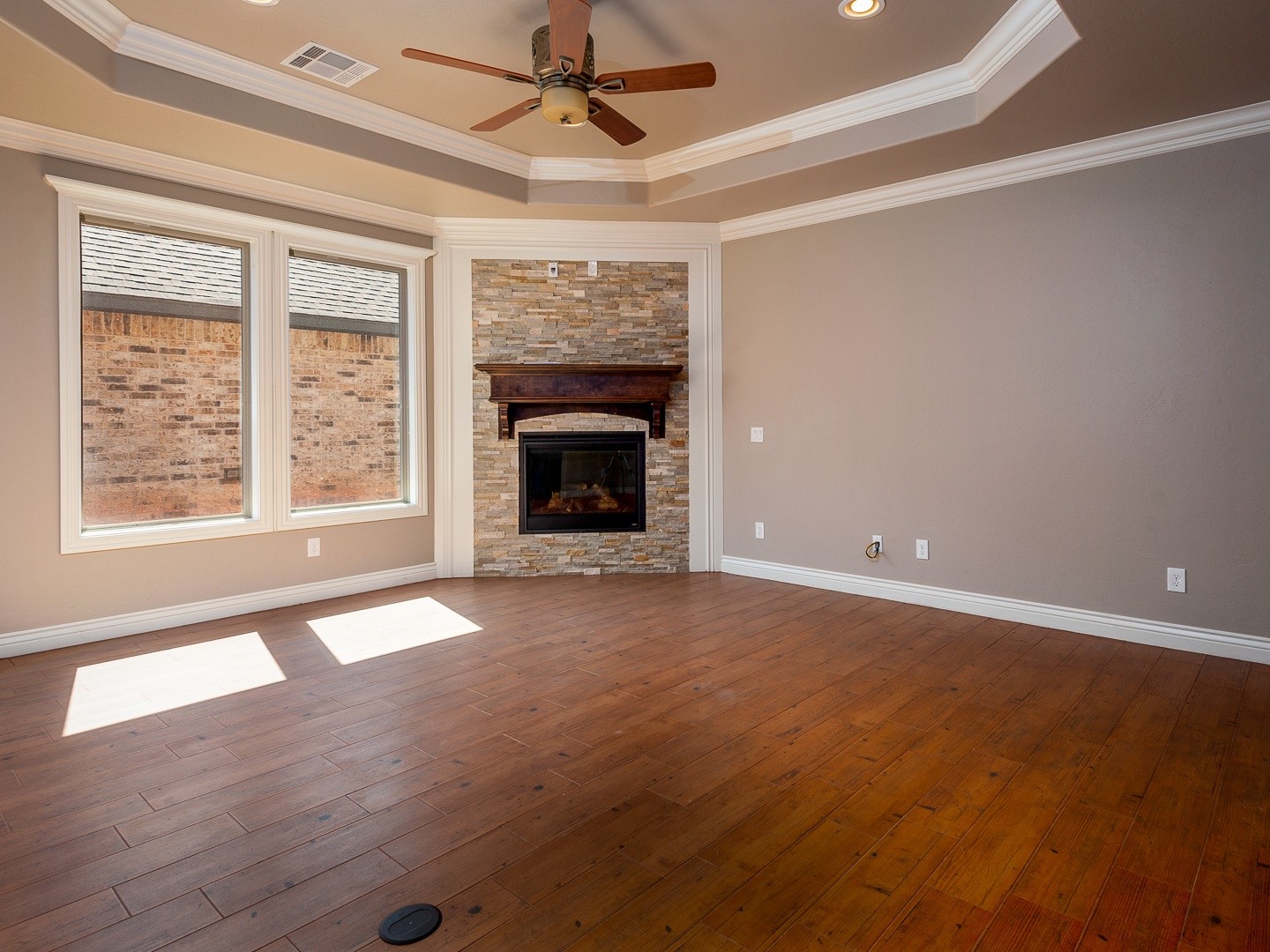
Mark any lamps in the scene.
[539,82,589,126]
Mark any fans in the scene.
[401,0,716,147]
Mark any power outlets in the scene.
[588,261,597,276]
[1168,568,1185,593]
[873,535,883,554]
[756,522,765,539]
[307,537,321,559]
[916,539,929,560]
